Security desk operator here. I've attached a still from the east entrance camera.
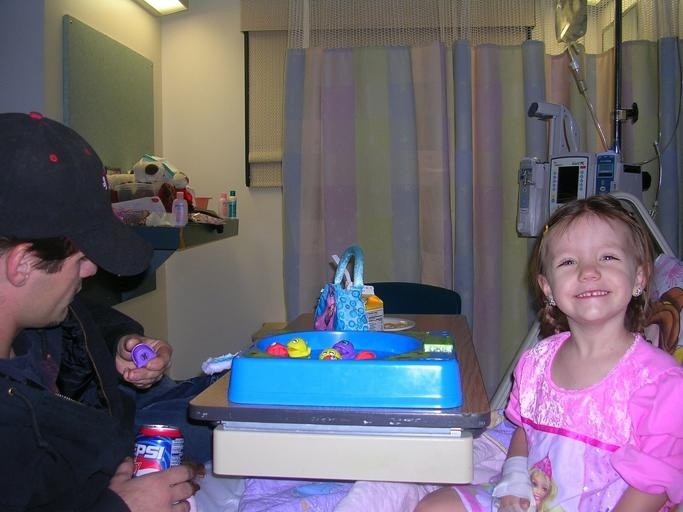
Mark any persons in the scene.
[527,453,559,512]
[402,192,681,512]
[0,108,202,511]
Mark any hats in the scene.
[0,112,153,280]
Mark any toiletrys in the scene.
[227,190,236,218]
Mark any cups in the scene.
[194,196,209,211]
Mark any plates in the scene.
[383,316,416,331]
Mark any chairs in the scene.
[361,282,462,316]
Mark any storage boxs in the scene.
[115,181,167,201]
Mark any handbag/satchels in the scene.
[312,242,373,332]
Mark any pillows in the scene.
[629,254,683,364]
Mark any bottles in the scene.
[228,190,238,219]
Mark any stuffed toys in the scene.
[158,171,192,210]
[104,153,175,187]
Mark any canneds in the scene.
[132,425,184,479]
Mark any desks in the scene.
[187,317,490,486]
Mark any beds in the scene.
[136,307,683,512]
